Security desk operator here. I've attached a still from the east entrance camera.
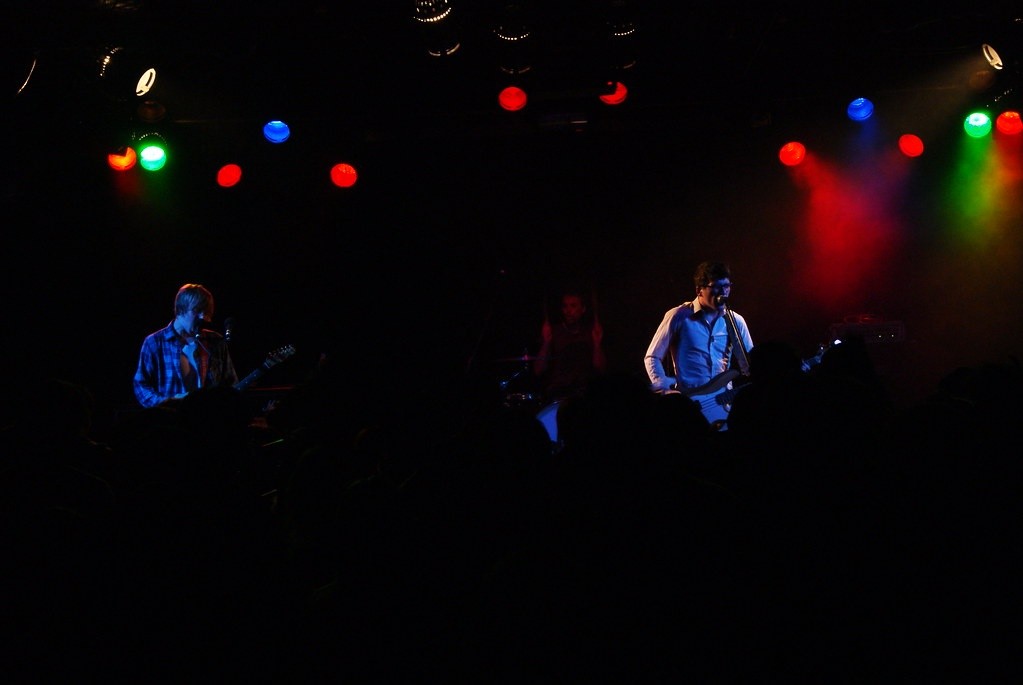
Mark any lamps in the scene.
[0,0,1023,193]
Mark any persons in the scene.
[643,260,752,430]
[536,291,602,394]
[133,282,237,410]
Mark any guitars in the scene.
[232,343,297,389]
[686,355,822,430]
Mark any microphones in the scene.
[224,318,235,341]
[720,296,731,309]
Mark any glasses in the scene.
[699,282,734,290]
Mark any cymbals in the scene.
[492,354,546,361]
